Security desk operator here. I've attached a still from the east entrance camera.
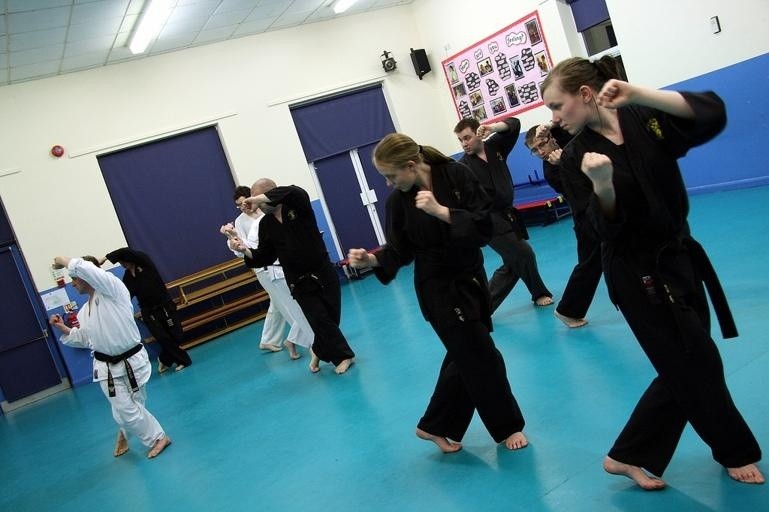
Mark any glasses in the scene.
[530,137,550,157]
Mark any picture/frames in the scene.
[445,17,550,116]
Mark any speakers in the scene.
[410,48,432,77]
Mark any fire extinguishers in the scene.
[62,304,87,351]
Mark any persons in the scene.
[540,55,548,71]
[229,176,357,375]
[538,53,768,492]
[46,254,173,459]
[522,120,603,330]
[346,132,528,455]
[448,65,458,83]
[219,185,316,360]
[479,64,488,76]
[98,247,194,372]
[484,60,493,74]
[452,115,555,317]
[514,60,524,77]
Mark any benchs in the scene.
[136,257,270,350]
